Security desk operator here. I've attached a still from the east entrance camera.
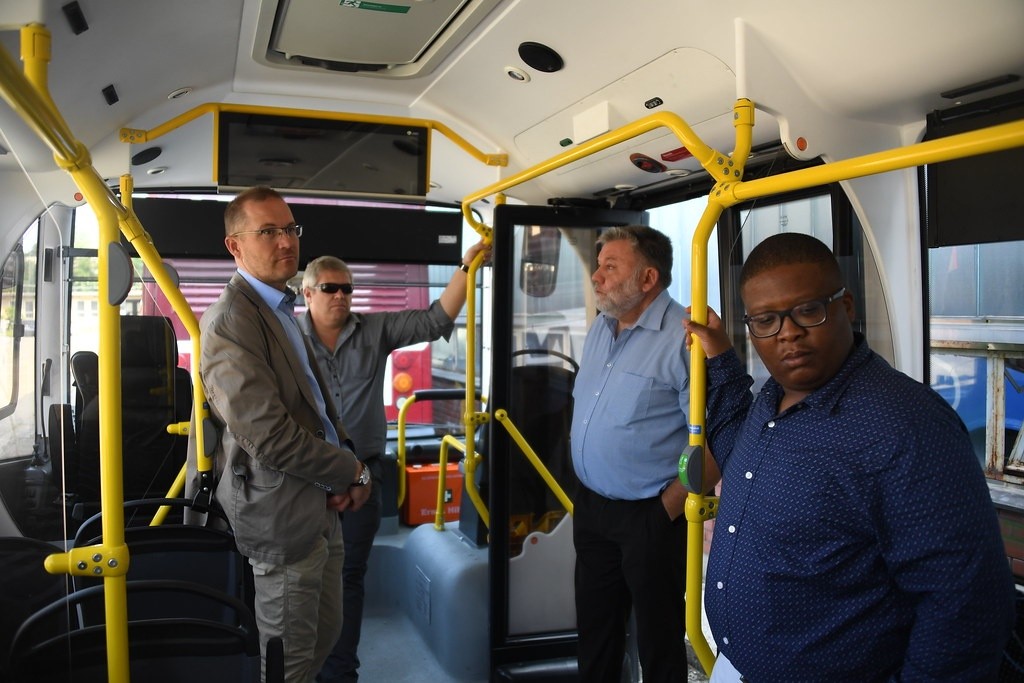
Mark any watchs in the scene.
[349,462,371,487]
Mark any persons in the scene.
[568,224,708,679]
[291,241,494,683]
[682,231,1024,683]
[196,185,372,682]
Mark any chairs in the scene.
[5,310,262,683]
[455,346,580,547]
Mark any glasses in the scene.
[307,282,354,295]
[743,288,847,338]
[230,225,303,240]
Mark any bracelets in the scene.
[458,260,469,274]
